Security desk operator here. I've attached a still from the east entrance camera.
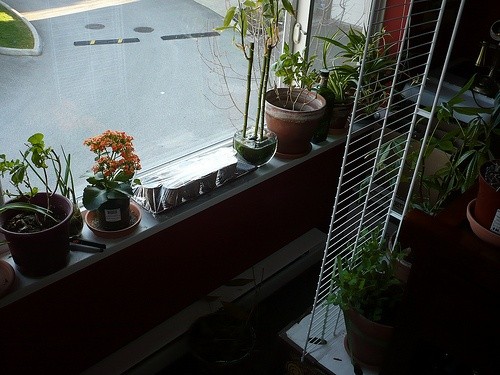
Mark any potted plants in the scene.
[0,133,83,278]
[357,72,500,279]
[213,0,399,168]
[323,222,412,368]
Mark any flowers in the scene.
[81,130,143,212]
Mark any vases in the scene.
[185,314,257,375]
[476,160,500,235]
[97,197,130,230]
